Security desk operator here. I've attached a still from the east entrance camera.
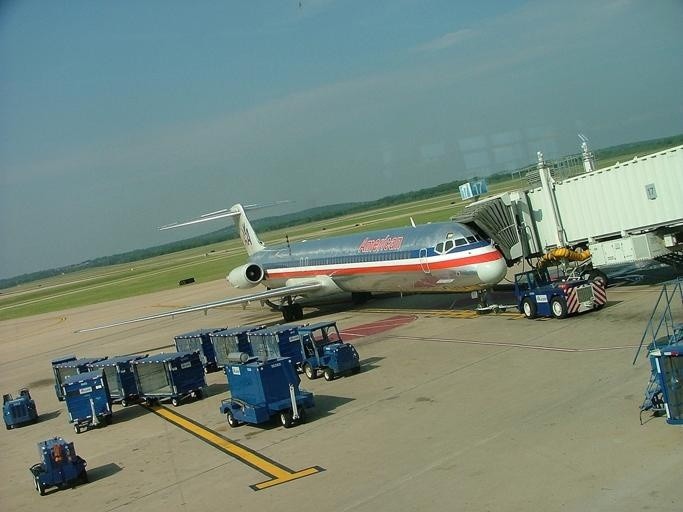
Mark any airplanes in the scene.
[83,201,512,333]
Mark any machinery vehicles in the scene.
[513,266,608,319]
